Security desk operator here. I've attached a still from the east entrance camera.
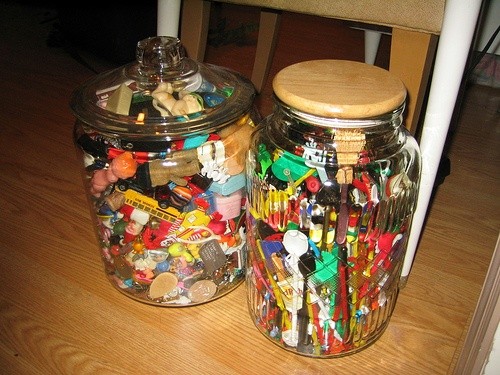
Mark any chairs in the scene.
[181,0,447,137]
